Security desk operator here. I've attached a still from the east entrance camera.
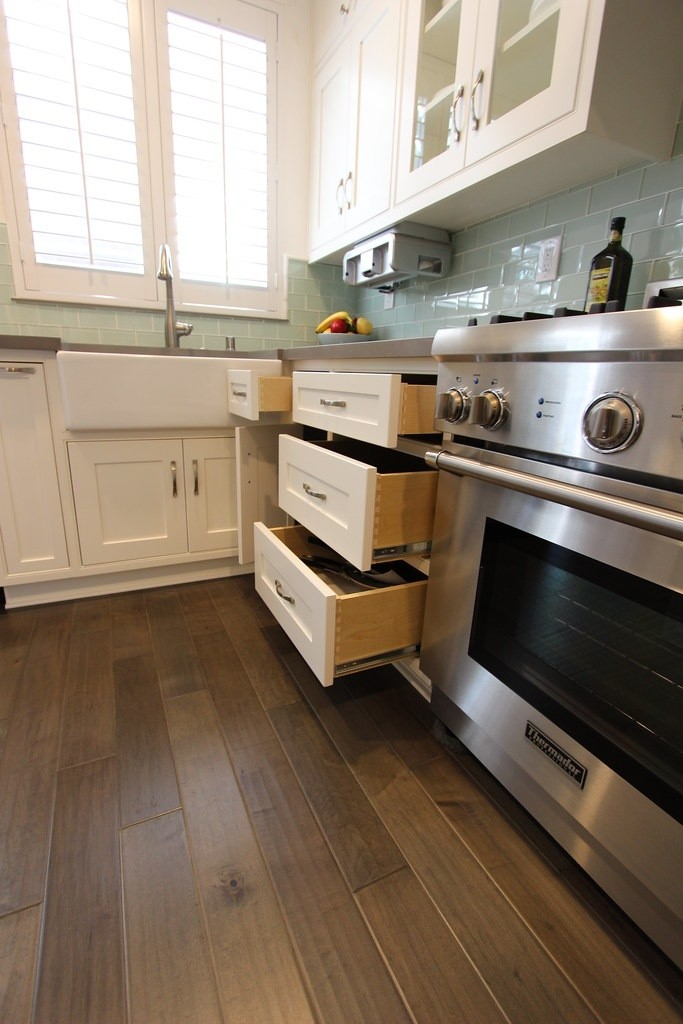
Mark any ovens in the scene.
[423,360,682,978]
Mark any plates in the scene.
[318,332,371,344]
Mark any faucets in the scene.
[156,243,194,348]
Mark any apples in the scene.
[330,319,346,332]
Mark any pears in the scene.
[356,317,371,334]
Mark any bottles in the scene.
[584,213,634,314]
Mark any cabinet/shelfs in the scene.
[65,427,244,584]
[227,338,433,705]
[1,334,80,610]
[398,0,683,229]
[309,1,398,268]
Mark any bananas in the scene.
[314,310,352,333]
